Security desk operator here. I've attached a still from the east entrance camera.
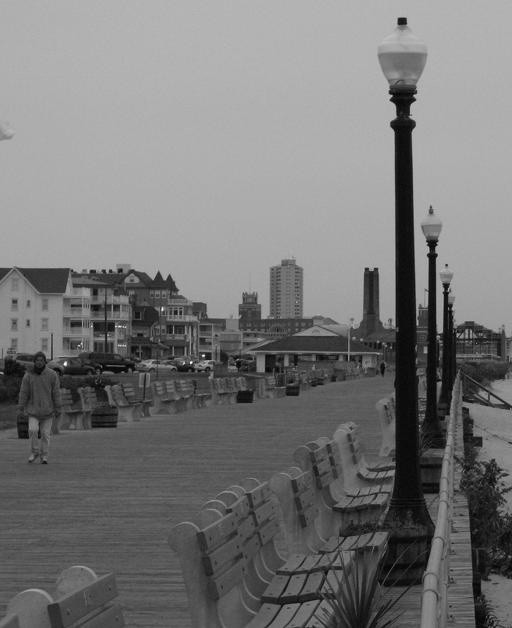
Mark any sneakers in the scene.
[28,454,38,462]
[40,455,48,464]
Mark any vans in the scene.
[79,351,133,374]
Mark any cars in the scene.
[134,355,217,372]
[45,356,104,376]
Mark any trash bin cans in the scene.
[17,414,41,439]
[91,406,118,427]
[237,390,253,403]
[286,386,299,396]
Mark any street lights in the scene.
[420,204,446,449]
[376,16,437,589]
[439,263,457,401]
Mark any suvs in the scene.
[9,353,35,371]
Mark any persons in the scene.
[18,352,63,464]
[380,360,386,376]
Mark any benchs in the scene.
[1,565,128,627]
[169,471,387,627]
[291,421,399,559]
[255,367,366,399]
[51,367,249,430]
[376,369,427,460]
